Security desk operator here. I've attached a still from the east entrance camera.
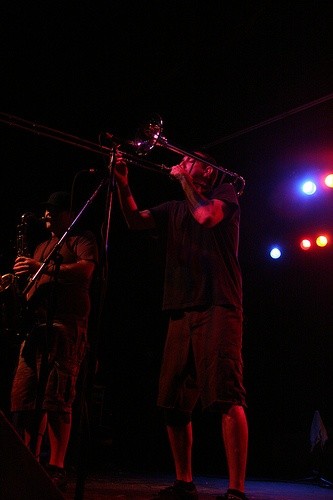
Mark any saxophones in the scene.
[0,212,48,337]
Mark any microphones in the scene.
[90,167,110,177]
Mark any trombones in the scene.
[0,112,246,197]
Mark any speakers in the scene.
[0,410,64,500]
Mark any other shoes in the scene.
[50,470,68,488]
[216,489,246,500]
[153,483,198,500]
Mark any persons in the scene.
[109,154,248,500]
[0,193,98,495]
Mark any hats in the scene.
[40,192,69,209]
